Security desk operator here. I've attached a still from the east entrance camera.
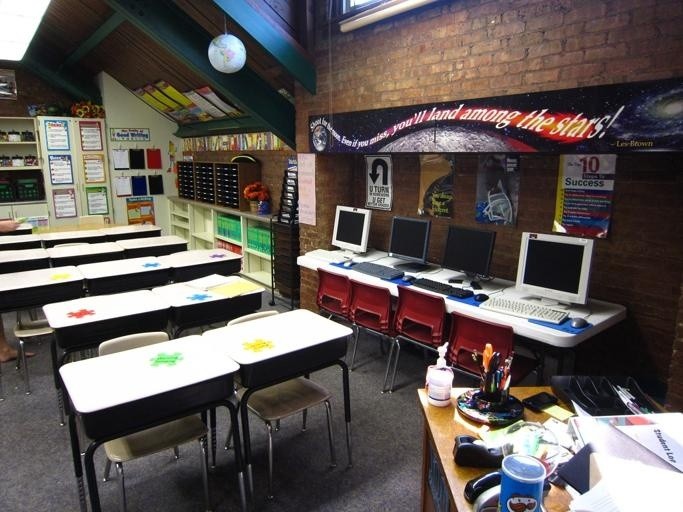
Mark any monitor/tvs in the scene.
[390,213,431,272]
[329,202,374,259]
[443,222,497,290]
[516,231,596,309]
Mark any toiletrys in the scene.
[426,342,454,408]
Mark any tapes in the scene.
[471,440,489,450]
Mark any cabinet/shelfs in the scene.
[0,116,48,204]
[168,195,278,290]
[175,159,260,211]
[38,116,114,223]
[0,204,48,226]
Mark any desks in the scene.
[293,237,629,348]
[411,372,668,512]
[1,221,356,512]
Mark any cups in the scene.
[480,389,511,403]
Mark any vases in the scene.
[249,200,258,214]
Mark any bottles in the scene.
[498,454,547,512]
[0,128,36,143]
[0,154,39,166]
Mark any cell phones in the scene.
[523,391,559,413]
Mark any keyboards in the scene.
[412,277,476,299]
[479,296,570,326]
[304,248,350,264]
[352,261,404,281]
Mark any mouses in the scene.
[571,317,588,330]
[344,260,353,267]
[474,293,490,304]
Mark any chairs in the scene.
[442,315,533,390]
[346,278,394,395]
[306,265,352,370]
[382,282,446,395]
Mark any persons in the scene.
[0,219,36,362]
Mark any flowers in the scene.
[242,179,269,201]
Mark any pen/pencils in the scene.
[470,341,517,391]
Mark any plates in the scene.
[456,388,524,425]
